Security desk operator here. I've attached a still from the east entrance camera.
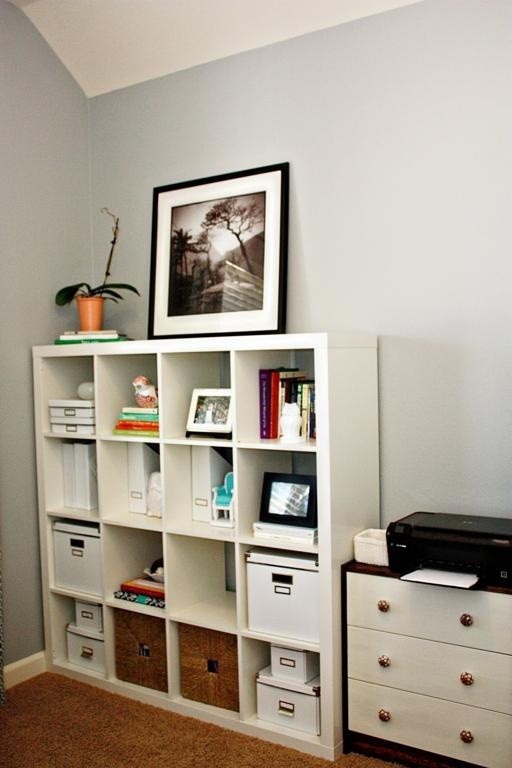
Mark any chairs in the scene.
[208,470,235,527]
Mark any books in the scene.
[259,366,316,440]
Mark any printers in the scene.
[384,509,512,589]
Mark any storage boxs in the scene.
[44,520,110,675]
[243,545,323,644]
[49,415,97,438]
[252,662,324,738]
[47,396,97,417]
[266,639,323,686]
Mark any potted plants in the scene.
[55,203,141,333]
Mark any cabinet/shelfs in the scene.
[339,552,512,768]
[29,330,382,763]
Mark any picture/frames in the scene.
[257,469,319,530]
[147,161,293,339]
[184,385,235,438]
